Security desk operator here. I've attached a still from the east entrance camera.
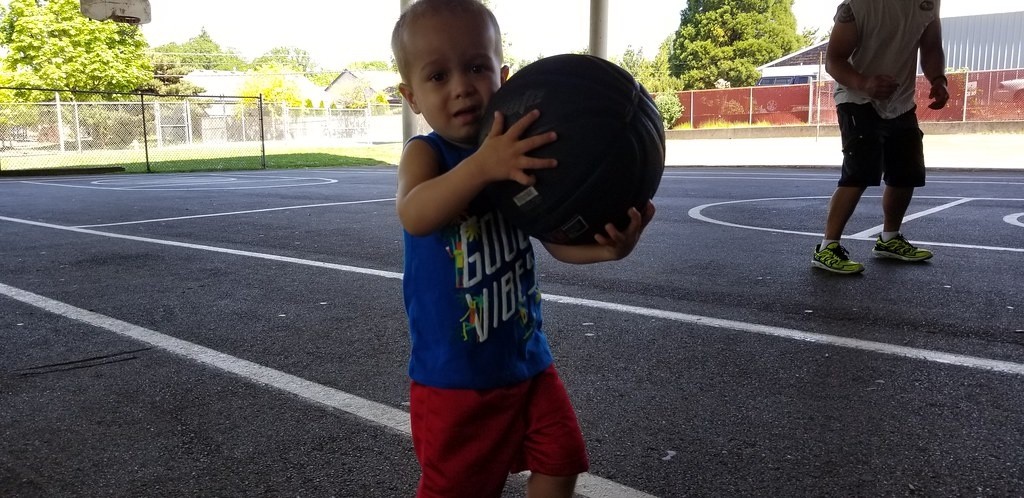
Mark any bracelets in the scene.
[931,74,948,86]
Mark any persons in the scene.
[392,0,656,498]
[811,0,949,274]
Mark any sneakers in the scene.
[871,232,934,262]
[811,242,865,274]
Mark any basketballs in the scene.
[476,54,665,245]
